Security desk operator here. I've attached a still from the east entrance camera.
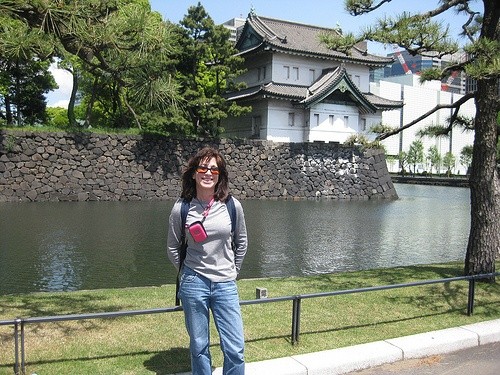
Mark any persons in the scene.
[165,147,249,375]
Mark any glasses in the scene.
[195,166,221,174]
